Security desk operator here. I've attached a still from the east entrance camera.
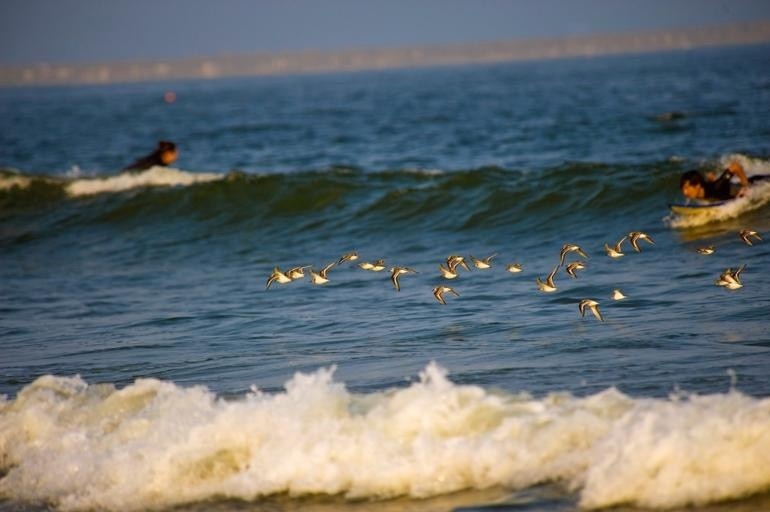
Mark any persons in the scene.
[679,159,770,205]
[126,138,179,172]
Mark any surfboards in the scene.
[669,200,732,215]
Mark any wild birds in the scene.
[505,263,525,272]
[713,263,747,290]
[695,245,719,255]
[387,266,419,292]
[739,229,763,247]
[438,253,497,280]
[535,231,656,323]
[266,251,387,290]
[431,285,460,305]
